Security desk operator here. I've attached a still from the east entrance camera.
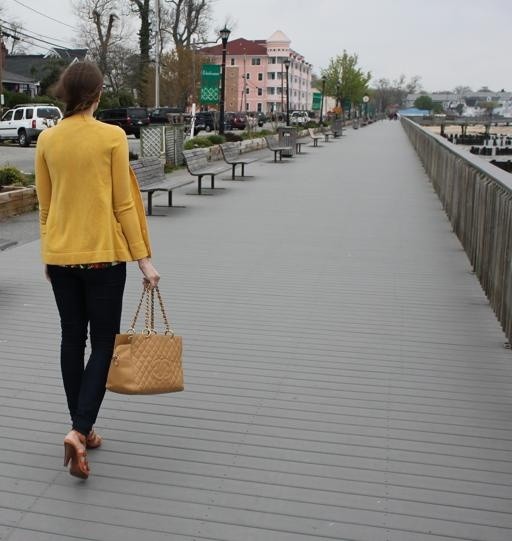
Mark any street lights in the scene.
[336,81,340,120]
[220,24,231,135]
[318,74,327,126]
[284,56,291,128]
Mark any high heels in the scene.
[64,429,91,480]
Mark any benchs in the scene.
[264,125,335,164]
[354,111,383,130]
[132,141,258,216]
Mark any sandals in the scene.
[85,427,102,449]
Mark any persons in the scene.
[31,59,161,483]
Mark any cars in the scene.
[96,107,311,138]
[0,104,64,147]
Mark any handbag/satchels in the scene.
[105,283,184,395]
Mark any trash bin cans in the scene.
[333,120,342,135]
[278,127,296,157]
[352,117,359,129]
